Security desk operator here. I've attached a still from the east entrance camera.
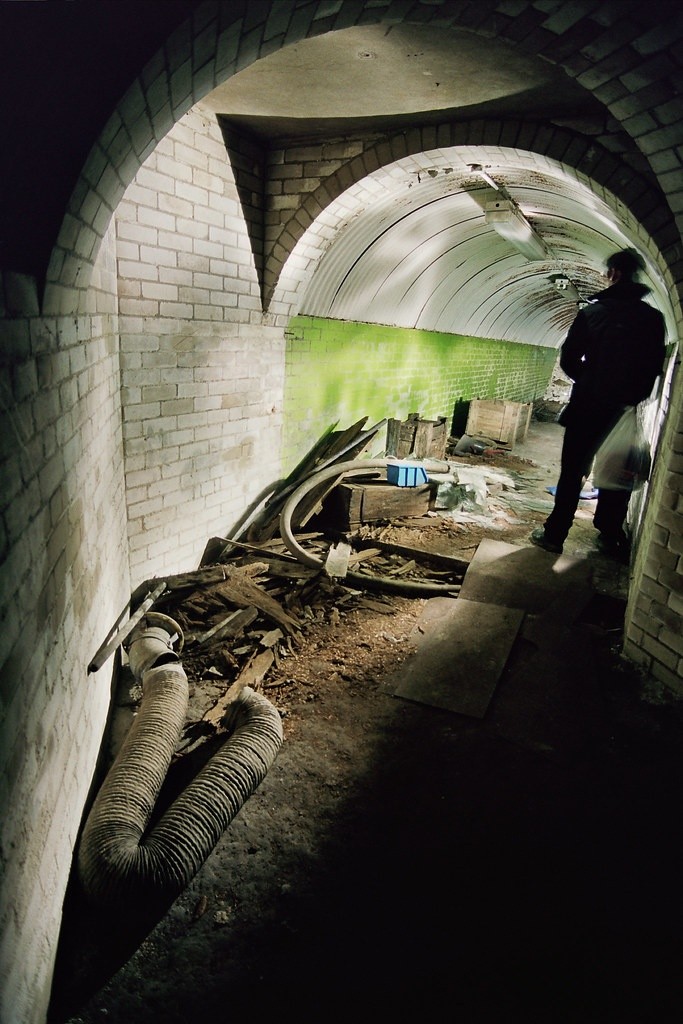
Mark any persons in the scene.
[530,248,666,553]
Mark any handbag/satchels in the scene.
[593,403,652,491]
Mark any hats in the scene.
[607,251,639,272]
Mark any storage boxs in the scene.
[465,398,534,451]
[387,413,449,460]
[334,474,445,524]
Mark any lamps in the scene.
[552,279,579,300]
[485,200,546,261]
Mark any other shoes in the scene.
[594,537,614,551]
[530,528,563,554]
